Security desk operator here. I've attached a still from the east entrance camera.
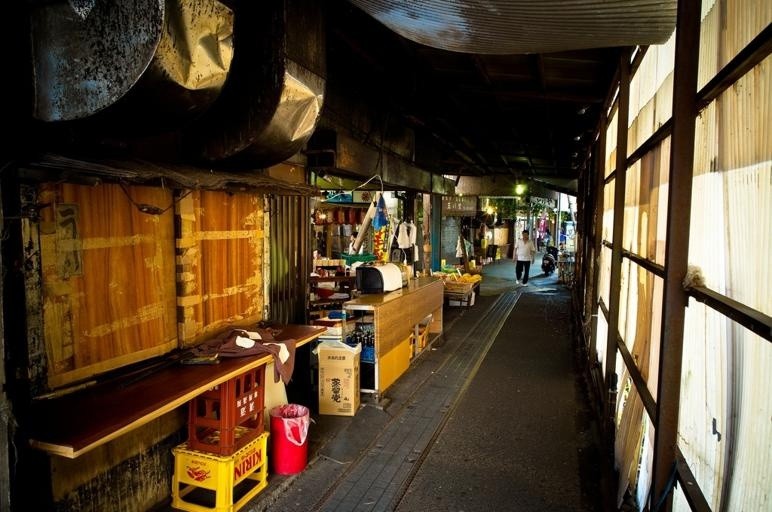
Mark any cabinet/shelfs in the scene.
[308,277,355,305]
[342,277,444,410]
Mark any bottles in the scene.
[350,326,374,346]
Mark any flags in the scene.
[372,194,390,232]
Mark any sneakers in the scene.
[516,279,528,287]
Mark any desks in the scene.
[444,281,480,314]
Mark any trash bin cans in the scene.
[269,404,309,475]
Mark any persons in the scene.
[347,232,364,255]
[323,231,329,248]
[512,230,535,288]
[313,249,329,260]
[317,231,324,248]
[311,230,318,247]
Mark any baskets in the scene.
[446,282,472,293]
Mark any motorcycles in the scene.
[541,246,559,276]
